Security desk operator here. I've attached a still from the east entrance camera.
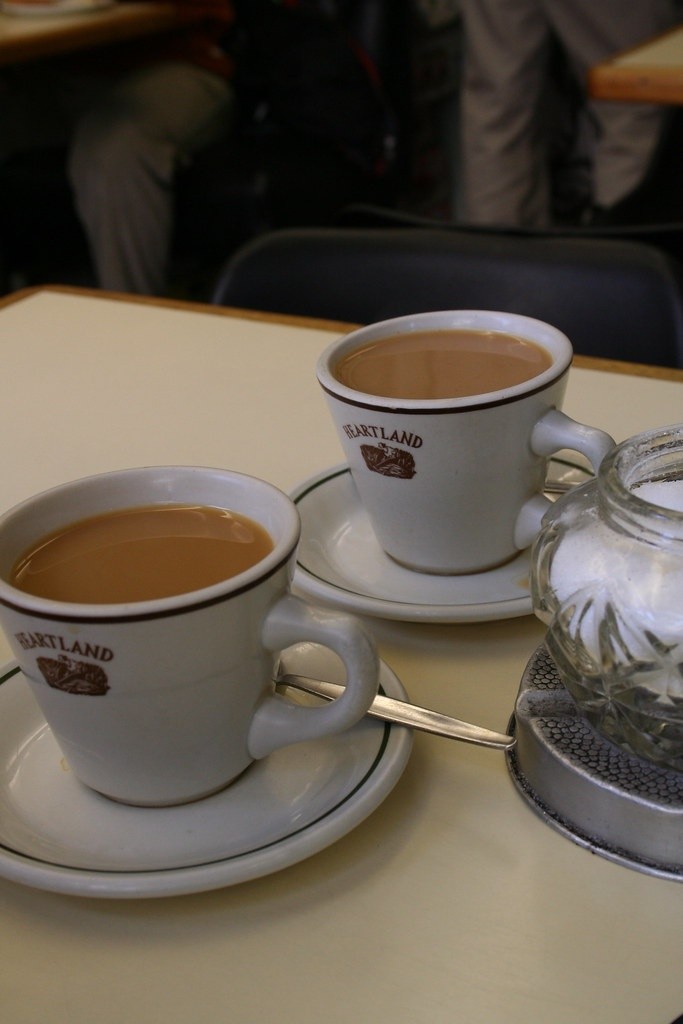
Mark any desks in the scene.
[0,286,680,1019]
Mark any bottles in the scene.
[533,424,682,775]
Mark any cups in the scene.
[318,311,617,574]
[0,466,380,809]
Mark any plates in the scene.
[289,457,596,622]
[1,654,414,898]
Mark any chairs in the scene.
[211,224,679,368]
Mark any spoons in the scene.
[276,660,516,751]
